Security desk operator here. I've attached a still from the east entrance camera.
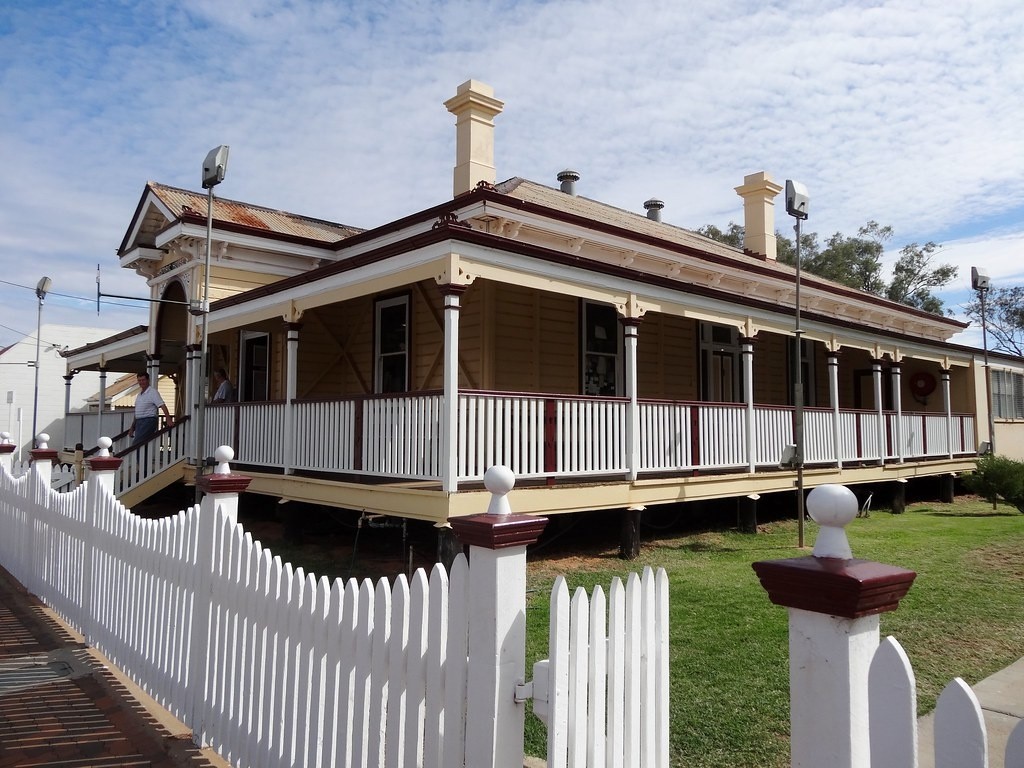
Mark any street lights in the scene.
[31,277,52,447]
[971,266,998,509]
[785,178,809,547]
[194,144,230,506]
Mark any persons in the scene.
[127,370,175,477]
[210,367,235,404]
